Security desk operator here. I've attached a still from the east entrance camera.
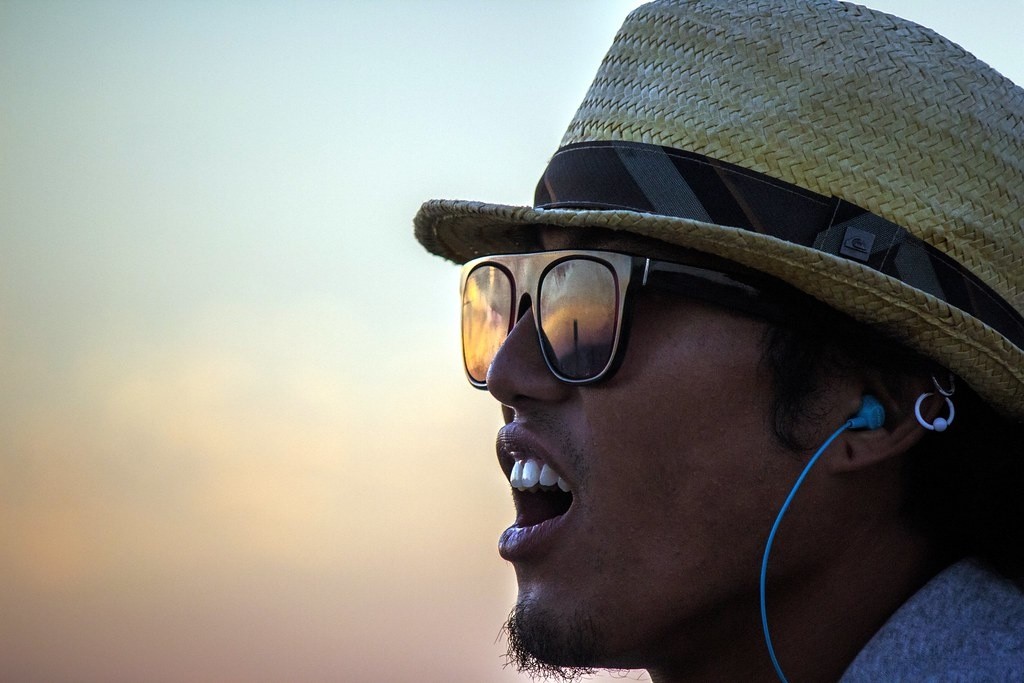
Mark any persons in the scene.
[412,0,1024,683]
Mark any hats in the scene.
[412,0,1024,422]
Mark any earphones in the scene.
[848,392,886,430]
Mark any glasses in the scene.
[460,249,866,390]
